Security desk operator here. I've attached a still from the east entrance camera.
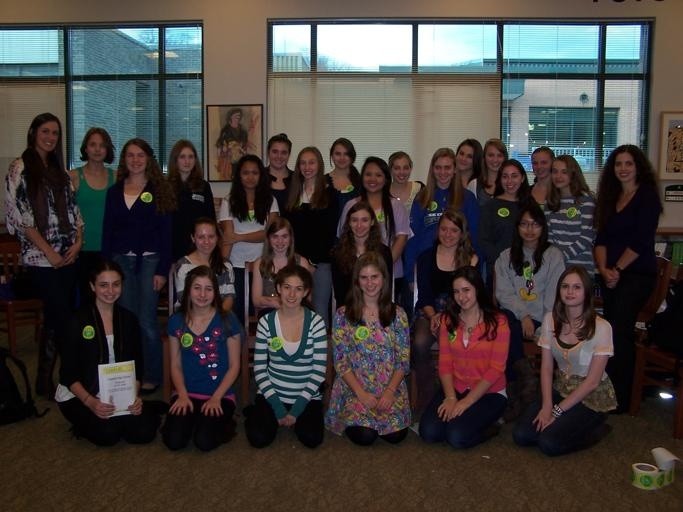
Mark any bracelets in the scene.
[612,263,623,275]
[386,386,395,393]
[443,395,457,401]
[553,404,566,415]
[551,409,560,419]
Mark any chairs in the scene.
[631,255,682,440]
[167,263,334,407]
[0,242,46,355]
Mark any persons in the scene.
[480,159,549,281]
[242,265,330,449]
[512,265,619,457]
[174,219,236,321]
[419,265,511,451]
[54,261,168,447]
[215,108,250,181]
[491,202,567,382]
[453,136,481,201]
[259,132,295,219]
[383,150,427,243]
[323,249,413,445]
[157,265,245,452]
[404,148,485,297]
[541,155,598,292]
[280,145,341,331]
[337,157,412,311]
[330,202,392,312]
[323,138,365,243]
[99,137,173,397]
[160,139,218,288]
[412,206,480,414]
[217,153,281,338]
[5,113,86,400]
[590,144,658,416]
[523,145,557,214]
[251,218,315,327]
[67,126,121,297]
[466,136,509,208]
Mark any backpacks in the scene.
[0,348,50,425]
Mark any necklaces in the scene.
[460,314,483,335]
[391,186,406,202]
[364,304,378,317]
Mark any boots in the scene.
[503,380,522,423]
[414,368,434,415]
[36,326,67,401]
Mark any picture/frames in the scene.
[205,104,263,182]
[656,111,682,182]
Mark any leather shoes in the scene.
[140,385,158,396]
